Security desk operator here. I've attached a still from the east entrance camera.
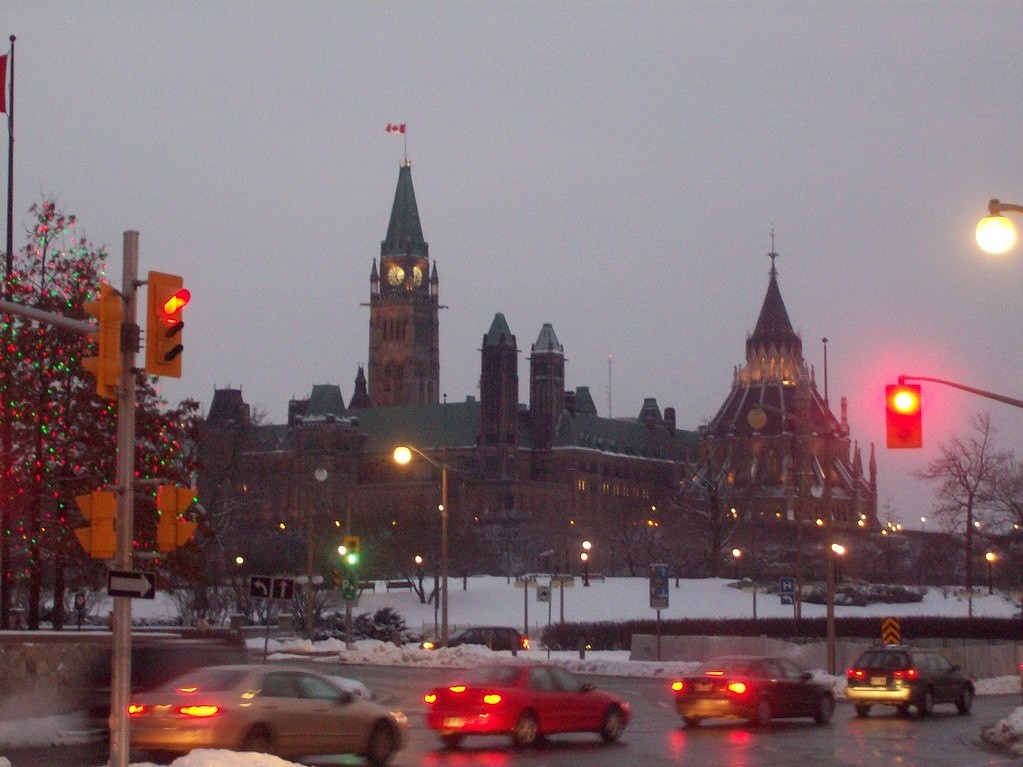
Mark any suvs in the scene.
[844,645,976,718]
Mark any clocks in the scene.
[412,265,422,286]
[387,264,405,286]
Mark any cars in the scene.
[673,654,837,730]
[125,664,404,762]
[447,626,530,651]
[424,661,636,748]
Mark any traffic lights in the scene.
[145,270,190,377]
[345,536,360,566]
[75,492,117,559]
[156,484,199,552]
[885,384,924,449]
[81,280,124,399]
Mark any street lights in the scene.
[986,551,996,595]
[732,547,742,581]
[920,517,926,534]
[582,540,591,587]
[830,543,841,584]
[392,440,453,648]
[836,546,845,585]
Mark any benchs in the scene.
[387,581,413,592]
[358,582,376,593]
[581,573,605,584]
[549,574,574,582]
